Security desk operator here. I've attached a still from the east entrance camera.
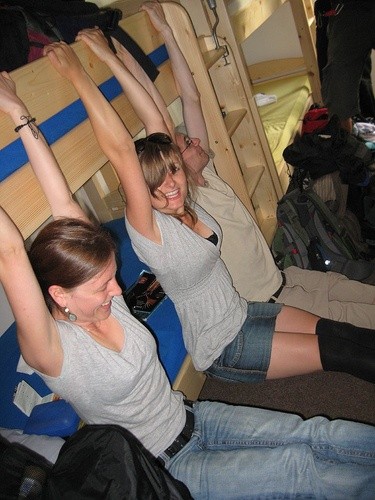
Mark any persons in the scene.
[0,72,375,500]
[42,28,374,384]
[74,0,375,329]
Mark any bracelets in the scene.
[14,116,36,132]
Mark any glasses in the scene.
[181,137,193,155]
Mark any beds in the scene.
[0,0,324,465]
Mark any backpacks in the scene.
[272,187,374,286]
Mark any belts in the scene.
[157,399,194,464]
[268,272,286,303]
